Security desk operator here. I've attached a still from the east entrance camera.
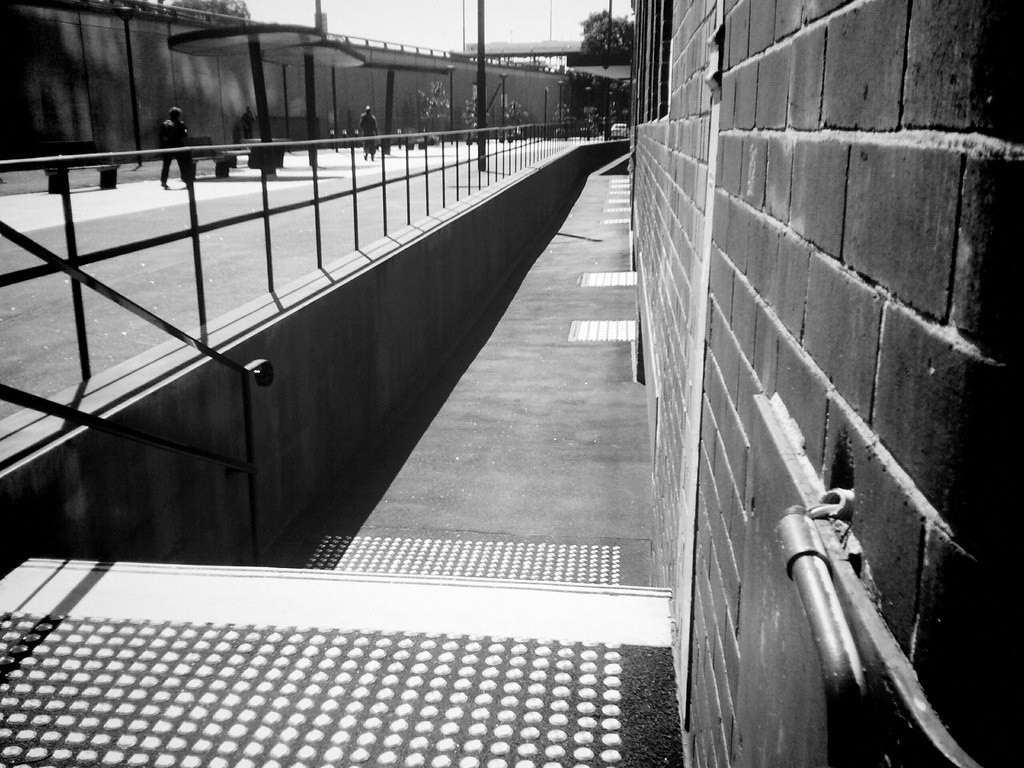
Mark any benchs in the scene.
[40,141,121,194]
[408,139,426,151]
[188,136,237,179]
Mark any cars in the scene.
[612,124,629,139]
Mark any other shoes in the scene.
[161,183,168,189]
[364,156,367,160]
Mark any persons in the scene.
[241,106,255,139]
[360,106,379,161]
[160,107,188,189]
